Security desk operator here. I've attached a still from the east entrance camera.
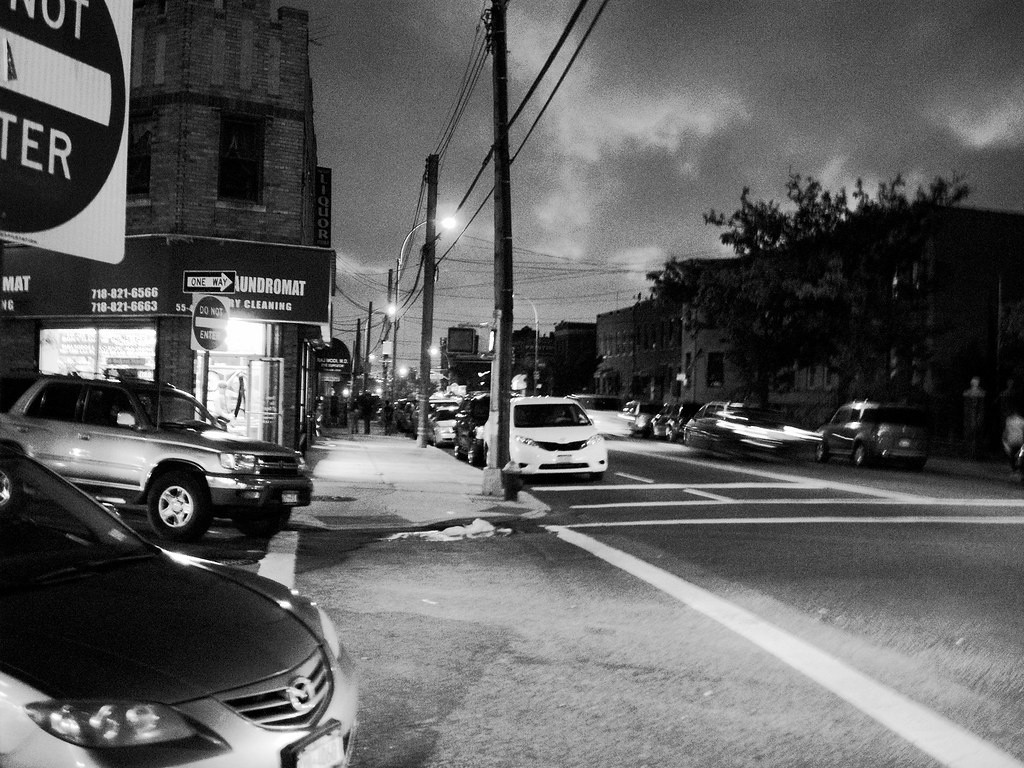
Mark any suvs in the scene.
[811,398,933,472]
[0,369,315,542]
[452,391,517,467]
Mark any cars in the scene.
[0,435,361,768]
[620,400,667,438]
[358,393,459,447]
[482,396,609,481]
[647,402,704,443]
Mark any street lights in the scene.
[427,345,451,385]
[389,217,456,422]
[512,293,538,395]
[363,305,396,361]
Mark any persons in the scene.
[347,388,399,440]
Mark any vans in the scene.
[683,402,751,448]
